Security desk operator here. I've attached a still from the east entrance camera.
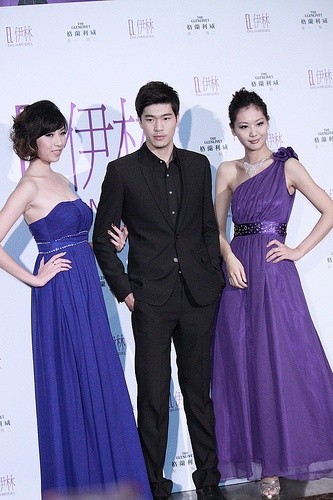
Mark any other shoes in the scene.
[196,486,227,500]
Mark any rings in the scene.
[52,261,56,266]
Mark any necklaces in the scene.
[242,153,273,178]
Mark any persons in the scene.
[214,87,333,499]
[92,81,225,500]
[0,100,154,500]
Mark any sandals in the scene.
[260,480,280,500]
